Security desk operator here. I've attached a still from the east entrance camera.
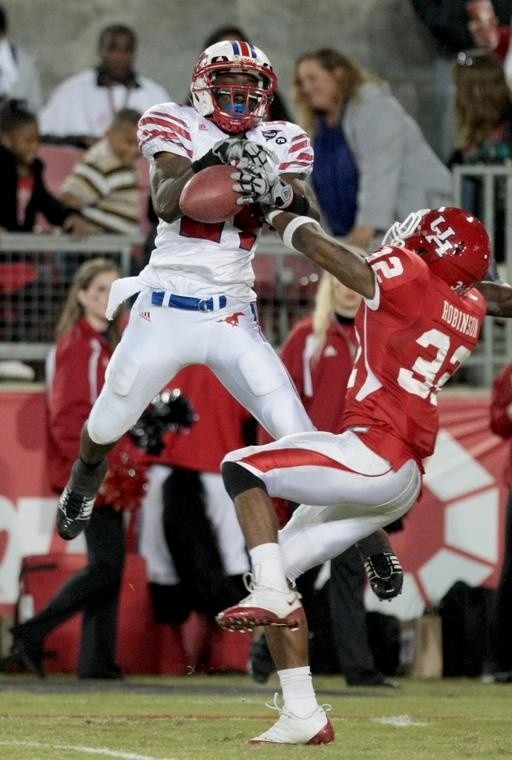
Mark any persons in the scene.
[0,0,510,746]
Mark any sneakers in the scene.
[356,530,403,599]
[56,459,108,540]
[217,585,304,632]
[248,707,335,745]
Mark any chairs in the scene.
[1,138,326,344]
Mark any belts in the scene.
[151,292,226,313]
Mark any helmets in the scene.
[401,207,492,296]
[190,41,278,133]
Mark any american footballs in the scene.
[180,163,246,221]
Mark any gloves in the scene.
[212,137,294,221]
